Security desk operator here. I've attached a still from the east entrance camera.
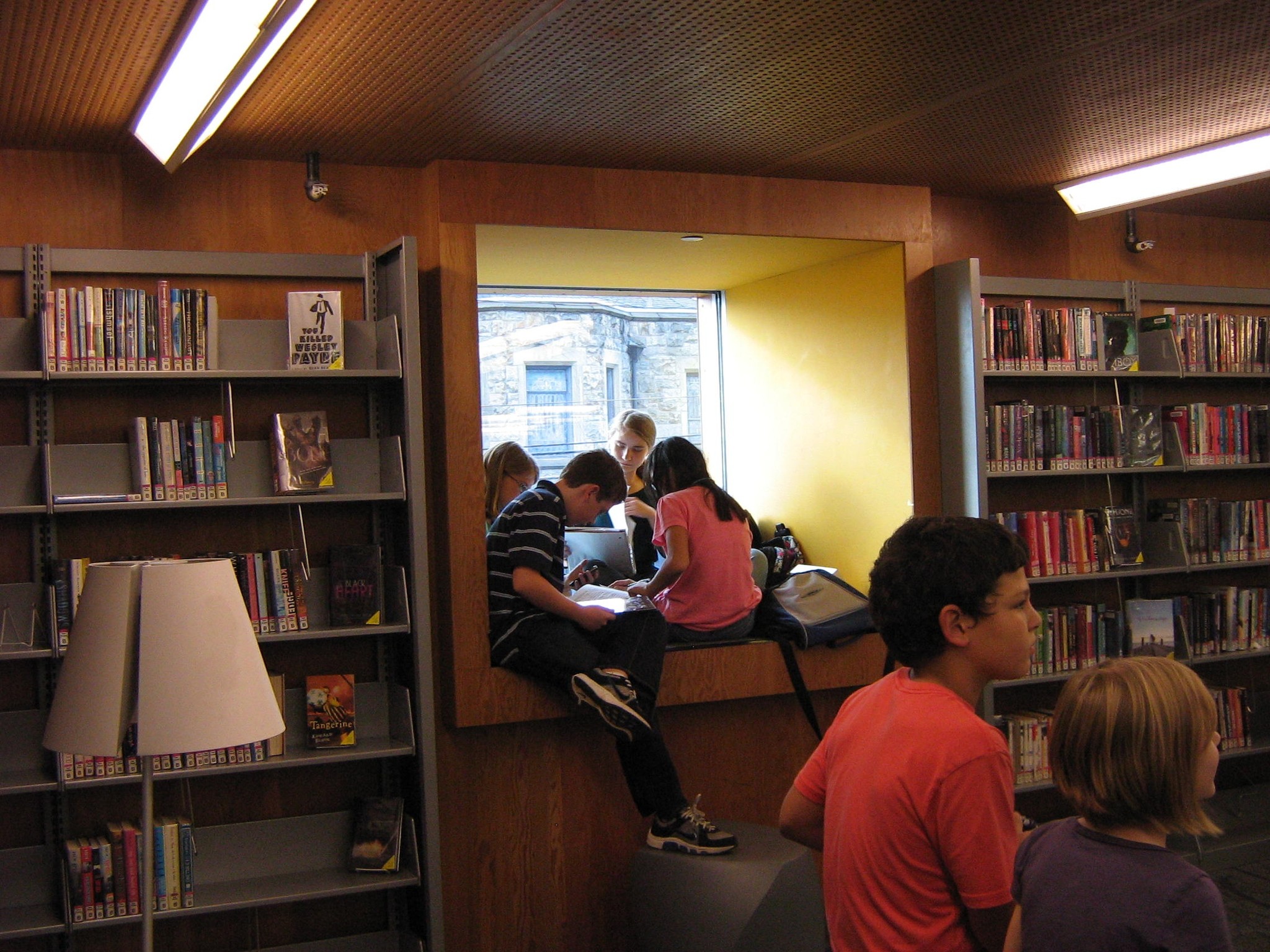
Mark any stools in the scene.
[624,814,833,952]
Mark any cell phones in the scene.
[569,564,600,588]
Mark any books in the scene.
[981,298,1270,786]
[570,584,630,614]
[49,549,309,651]
[54,493,142,504]
[288,291,344,371]
[61,675,287,780]
[350,796,406,872]
[127,415,228,501]
[46,280,218,373]
[269,410,335,495]
[328,545,386,626]
[65,816,194,922]
[565,486,636,578]
[305,674,357,748]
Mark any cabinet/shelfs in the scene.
[0,230,445,952]
[936,258,1270,794]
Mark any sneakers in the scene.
[645,794,738,856]
[571,668,653,743]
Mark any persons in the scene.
[608,436,762,642]
[780,513,1041,952]
[563,408,666,586]
[484,441,539,536]
[486,449,737,854]
[1004,656,1233,952]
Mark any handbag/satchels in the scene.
[759,569,879,650]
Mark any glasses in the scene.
[504,472,528,493]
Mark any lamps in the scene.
[1052,129,1270,230]
[129,0,326,177]
[39,559,287,951]
